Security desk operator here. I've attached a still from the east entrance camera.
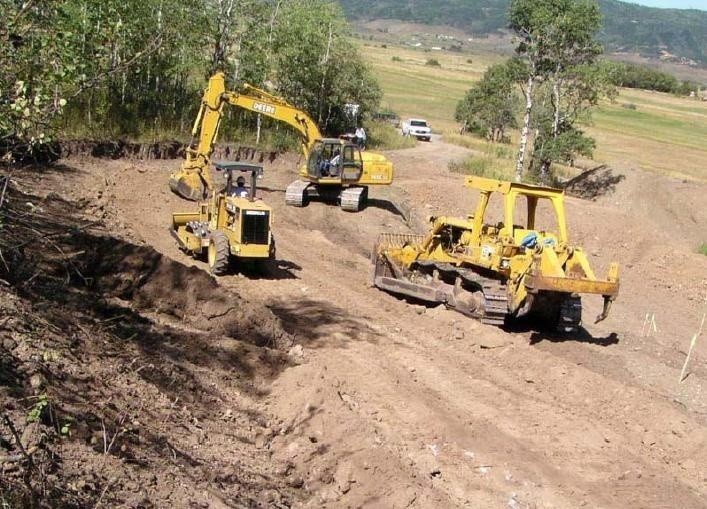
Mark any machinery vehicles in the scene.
[168,71,393,212]
[168,160,276,277]
[373,175,619,335]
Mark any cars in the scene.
[402,119,432,141]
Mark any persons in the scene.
[355,122,366,149]
[229,176,250,210]
[318,148,340,176]
